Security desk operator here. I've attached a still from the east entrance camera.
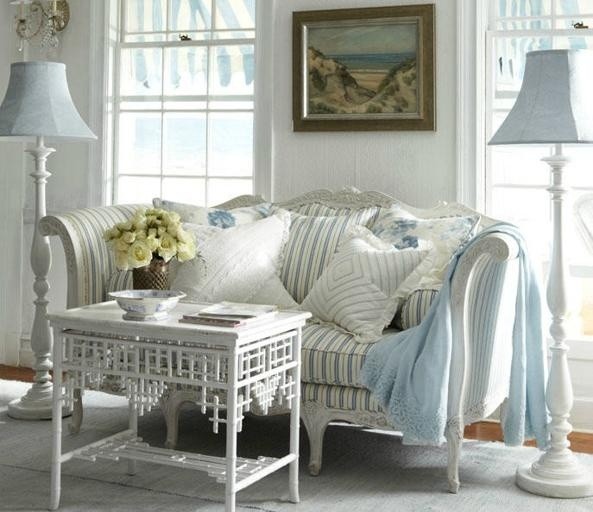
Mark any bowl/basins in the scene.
[106,288,187,316]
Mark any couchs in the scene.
[40,184,522,493]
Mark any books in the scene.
[181,300,279,326]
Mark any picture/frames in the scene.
[293,3,437,131]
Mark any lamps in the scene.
[10,1,70,61]
[486,50,593,499]
[1,61,97,421]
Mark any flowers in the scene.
[102,206,196,271]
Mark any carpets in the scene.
[1,378,593,511]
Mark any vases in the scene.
[133,258,170,290]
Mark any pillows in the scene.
[291,223,436,343]
[169,209,301,315]
[152,197,271,229]
[268,205,381,302]
[367,206,481,330]
